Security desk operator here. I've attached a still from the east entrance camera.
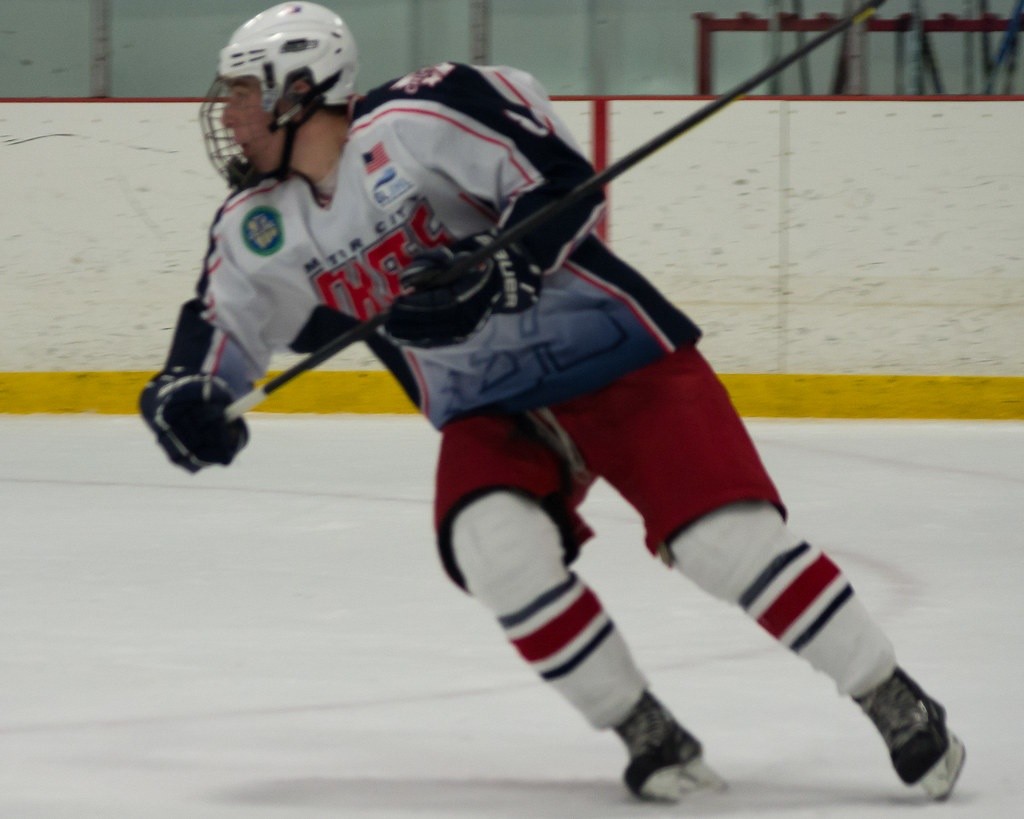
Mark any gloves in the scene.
[139,366,252,474]
[386,232,545,357]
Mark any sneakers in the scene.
[856,668,969,805]
[608,694,725,805]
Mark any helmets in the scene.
[193,2,357,182]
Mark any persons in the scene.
[139,0,969,809]
